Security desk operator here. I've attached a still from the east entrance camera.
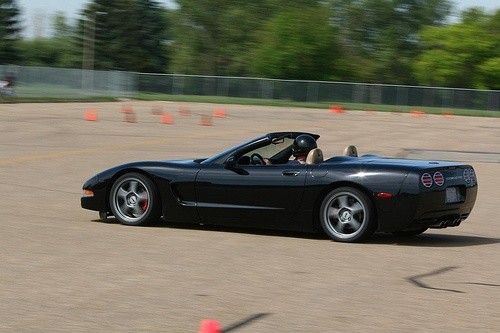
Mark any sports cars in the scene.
[78,128,480,245]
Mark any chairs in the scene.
[305,147,324,165]
[343,143,358,158]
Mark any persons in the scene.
[259,133,318,166]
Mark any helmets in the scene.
[292,134,317,156]
